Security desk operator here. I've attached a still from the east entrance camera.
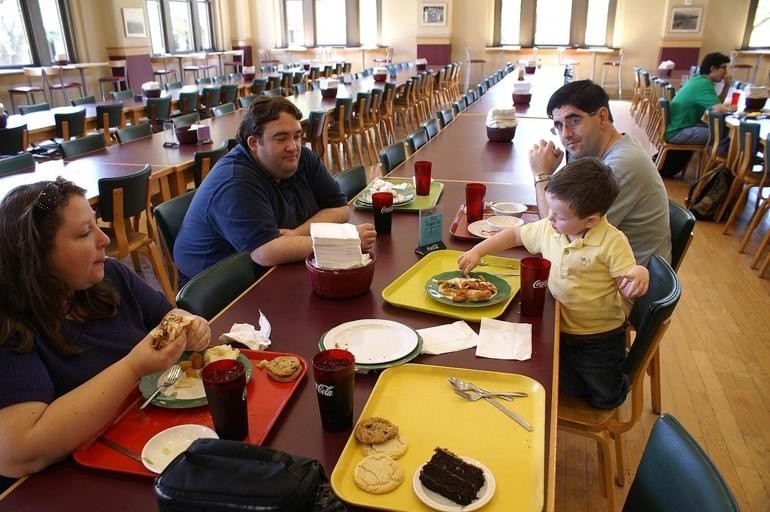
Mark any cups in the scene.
[415,160,432,196]
[200,357,249,441]
[518,255,552,316]
[310,349,355,434]
[371,192,393,235]
[731,92,741,105]
[464,183,488,226]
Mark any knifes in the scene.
[97,435,144,462]
[475,387,533,433]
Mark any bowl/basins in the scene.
[745,97,768,110]
[0,112,9,129]
[526,67,536,73]
[140,89,162,98]
[241,63,427,97]
[485,124,517,143]
[173,124,200,144]
[486,216,526,234]
[512,93,532,104]
[491,202,529,219]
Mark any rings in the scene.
[201,336,209,344]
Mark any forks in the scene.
[454,388,530,402]
[137,364,181,410]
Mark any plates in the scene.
[317,317,425,373]
[158,364,207,399]
[357,187,416,209]
[466,219,498,239]
[136,346,253,410]
[425,270,513,309]
[140,422,221,476]
[411,453,496,512]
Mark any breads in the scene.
[420,450,485,506]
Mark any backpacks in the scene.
[684,165,744,222]
[652,147,696,177]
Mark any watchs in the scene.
[534,173,553,187]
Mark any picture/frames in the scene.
[120,7,148,40]
[669,7,704,34]
[420,2,446,27]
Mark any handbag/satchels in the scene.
[153,436,346,511]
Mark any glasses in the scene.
[551,112,599,137]
[713,65,728,68]
[20,175,76,235]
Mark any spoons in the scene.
[447,376,514,403]
[478,260,519,270]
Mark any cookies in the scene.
[267,365,302,381]
[355,417,399,443]
[267,356,299,375]
[354,454,404,493]
[360,433,408,460]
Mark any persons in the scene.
[457,156,650,409]
[174,95,377,290]
[664,52,738,158]
[0,176,211,496]
[529,79,672,320]
